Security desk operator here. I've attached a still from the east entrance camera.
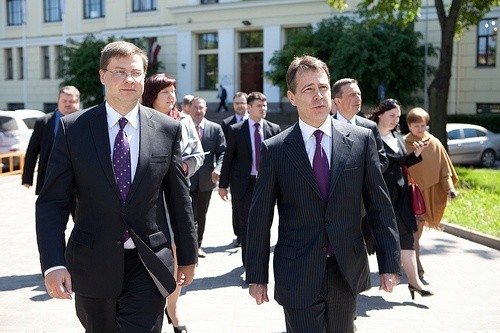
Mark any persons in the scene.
[34,41,202,333]
[22,73,459,333]
[246,56,403,333]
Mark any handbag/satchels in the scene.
[404,166,426,215]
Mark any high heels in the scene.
[165,306,187,333]
[408,282,434,300]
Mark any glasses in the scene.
[387,99,400,106]
[103,68,146,79]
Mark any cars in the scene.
[0,110,48,169]
[446,123,500,167]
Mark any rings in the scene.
[50,291,53,294]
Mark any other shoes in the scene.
[235,239,241,247]
[197,247,205,258]
[420,273,429,285]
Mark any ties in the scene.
[112,117,131,243]
[240,117,243,122]
[253,123,262,171]
[347,120,351,124]
[196,124,202,142]
[312,129,334,259]
